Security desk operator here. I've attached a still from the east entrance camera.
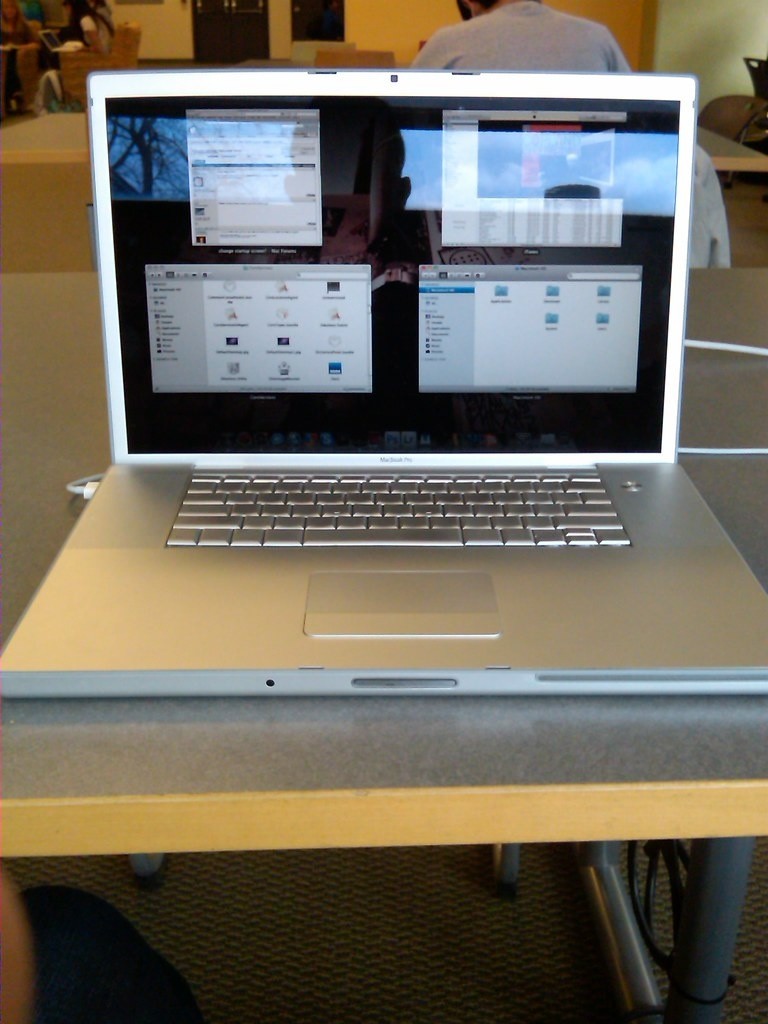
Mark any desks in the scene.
[0,112,768,173]
[0,270,768,1024]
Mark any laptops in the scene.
[0,67,768,697]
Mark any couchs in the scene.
[16,20,142,113]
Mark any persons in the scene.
[0,0,113,114]
[411,0,629,73]
[238,121,421,294]
[0,868,203,1024]
